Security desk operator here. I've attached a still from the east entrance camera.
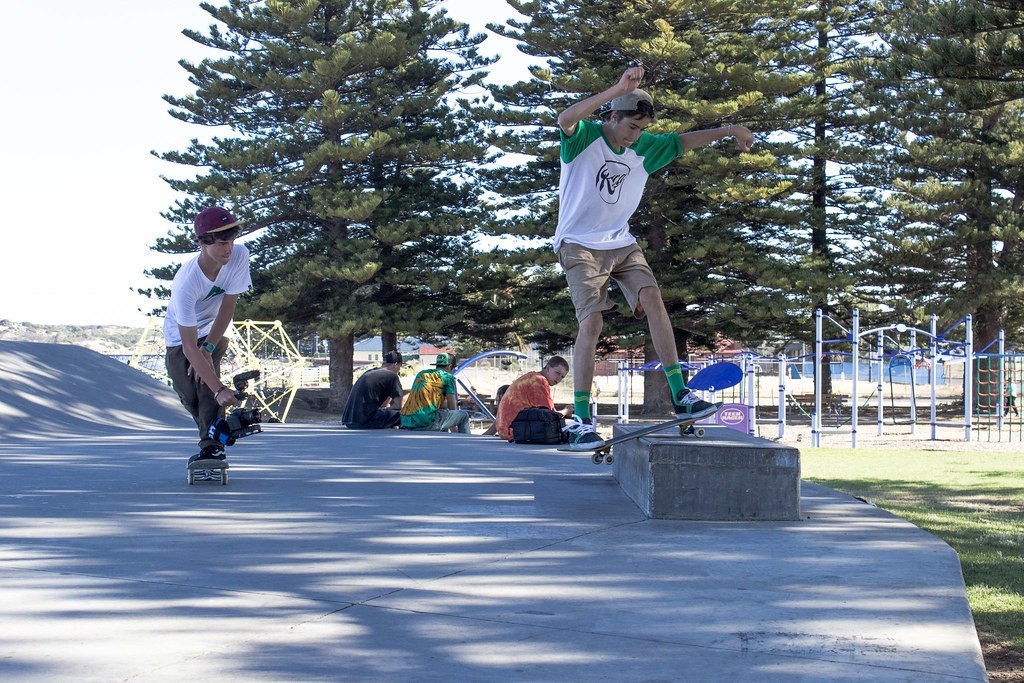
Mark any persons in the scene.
[341,351,409,429]
[400,352,471,433]
[555,64,757,451]
[493,356,574,443]
[163,206,255,460]
[1003,376,1020,419]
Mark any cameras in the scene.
[226,408,261,431]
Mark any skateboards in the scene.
[557,401,724,465]
[182,455,231,488]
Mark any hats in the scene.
[194,207,242,236]
[384,350,406,364]
[430,352,457,366]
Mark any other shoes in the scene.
[200,445,227,461]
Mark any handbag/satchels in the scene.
[506,404,567,445]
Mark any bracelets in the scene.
[728,124,733,138]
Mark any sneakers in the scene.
[569,413,604,449]
[670,389,718,416]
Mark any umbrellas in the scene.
[620,355,699,371]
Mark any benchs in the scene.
[458,394,496,415]
[786,394,850,417]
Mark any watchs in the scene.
[201,341,216,353]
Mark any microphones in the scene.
[233,370,260,382]
